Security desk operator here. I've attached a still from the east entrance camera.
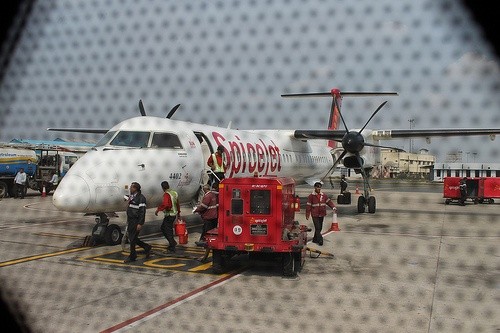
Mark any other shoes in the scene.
[312,238,325,245]
[145,245,152,255]
[168,241,177,248]
[124,256,138,263]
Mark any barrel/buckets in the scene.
[179,232,188,244]
[175,223,186,236]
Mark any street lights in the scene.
[407,118,416,153]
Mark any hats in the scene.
[217,146,224,152]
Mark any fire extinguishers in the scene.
[295,195,301,211]
[290,196,294,211]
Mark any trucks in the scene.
[0,144,80,199]
[442,176,500,205]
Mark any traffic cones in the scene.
[354,185,361,195]
[41,185,47,200]
[327,212,341,232]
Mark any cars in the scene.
[194,171,313,277]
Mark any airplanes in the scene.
[45,88,499,251]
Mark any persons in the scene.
[155,181,181,251]
[14,168,26,199]
[340,178,347,194]
[48,171,58,190]
[459,177,467,206]
[306,182,336,245]
[193,182,219,246]
[124,182,151,263]
